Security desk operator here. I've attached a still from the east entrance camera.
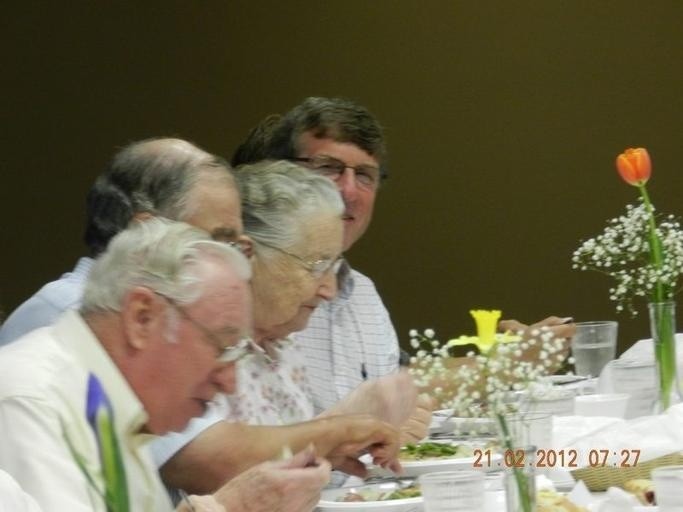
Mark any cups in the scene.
[611,357,659,417]
[421,471,484,512]
[504,411,555,471]
[574,393,630,419]
[569,321,619,379]
[651,466,683,512]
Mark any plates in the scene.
[514,375,590,399]
[357,442,504,475]
[431,408,501,435]
[314,479,423,511]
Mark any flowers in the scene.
[58,367,131,512]
[572,147,683,409]
[409,309,578,511]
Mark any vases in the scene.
[497,412,555,511]
[647,301,683,414]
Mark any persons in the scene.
[220,156,436,449]
[0,211,334,511]
[233,96,578,407]
[0,135,405,494]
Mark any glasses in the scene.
[138,284,252,366]
[278,153,387,186]
[248,235,345,280]
